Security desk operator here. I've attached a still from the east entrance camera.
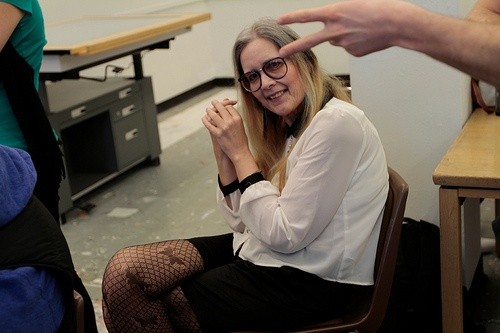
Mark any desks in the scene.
[432,109,500,333]
[38,26,191,83]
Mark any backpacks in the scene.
[385,218,484,332]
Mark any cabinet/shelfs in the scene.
[42,79,162,224]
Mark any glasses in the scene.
[238,57,288,92]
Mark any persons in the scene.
[0,0,97,333]
[276,0,500,88]
[101,16,389,333]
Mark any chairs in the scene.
[301,167,408,333]
